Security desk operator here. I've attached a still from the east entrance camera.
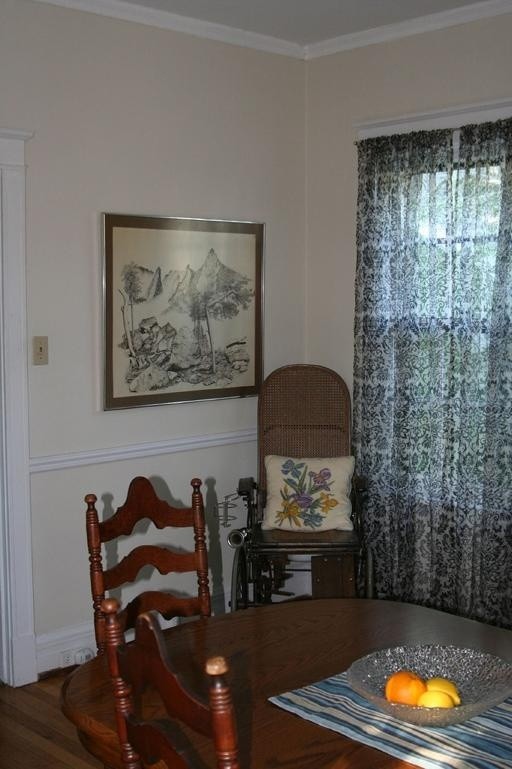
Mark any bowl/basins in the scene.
[346,643,512,725]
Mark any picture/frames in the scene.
[101,211,264,411]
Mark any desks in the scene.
[57,595,511,768]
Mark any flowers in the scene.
[274,458,338,529]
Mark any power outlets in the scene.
[60,647,94,668]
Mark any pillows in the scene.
[260,454,355,533]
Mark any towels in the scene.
[267,670,512,768]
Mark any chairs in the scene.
[84,476,211,654]
[100,598,241,768]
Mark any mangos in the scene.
[417,678,461,710]
